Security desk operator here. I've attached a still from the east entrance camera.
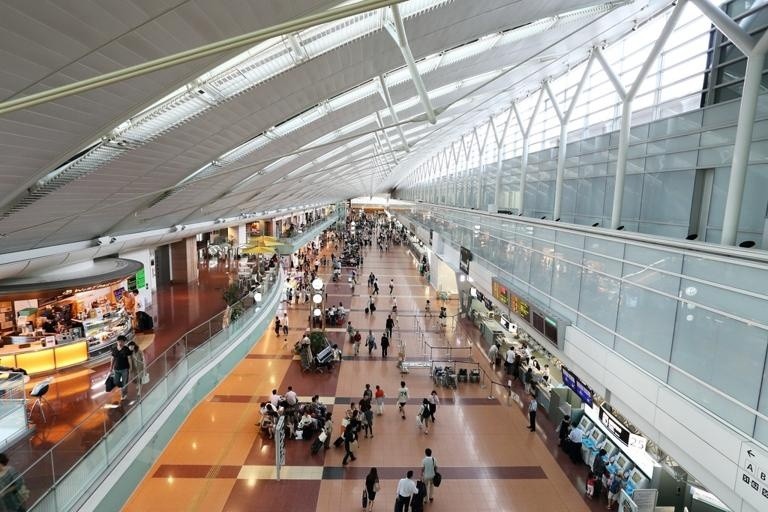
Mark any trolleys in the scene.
[433,366,480,387]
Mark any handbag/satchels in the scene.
[16,485,32,505]
[433,472,441,487]
[348,440,359,452]
[318,432,327,443]
[104,375,117,392]
[416,414,424,429]
[141,373,151,385]
[362,489,368,509]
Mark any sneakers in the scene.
[429,497,434,501]
[527,425,536,432]
[424,501,428,504]
[351,457,356,461]
[342,462,349,465]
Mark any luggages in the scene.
[310,438,323,454]
[333,437,345,448]
[495,357,502,365]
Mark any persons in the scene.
[521,342,531,366]
[126,341,147,398]
[524,366,534,395]
[259,381,438,465]
[108,334,134,402]
[41,314,60,337]
[422,255,428,276]
[565,420,590,465]
[288,210,407,269]
[425,299,446,328]
[487,341,501,365]
[527,395,538,433]
[505,346,519,376]
[202,238,232,273]
[365,466,379,512]
[233,252,279,304]
[584,448,630,510]
[419,447,439,506]
[555,414,572,449]
[541,364,554,388]
[275,259,397,360]
[393,469,419,511]
[530,355,541,372]
[0,453,28,512]
[122,289,153,331]
[86,301,99,320]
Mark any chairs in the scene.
[430,364,480,388]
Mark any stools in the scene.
[27,380,57,427]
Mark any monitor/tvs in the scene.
[113,287,125,303]
[616,456,626,467]
[592,430,600,440]
[576,377,593,409]
[580,419,588,428]
[476,291,483,302]
[603,443,612,454]
[561,365,577,394]
[485,298,492,311]
[631,472,642,483]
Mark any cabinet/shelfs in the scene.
[70,304,135,360]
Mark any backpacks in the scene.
[372,482,381,492]
[422,406,430,419]
